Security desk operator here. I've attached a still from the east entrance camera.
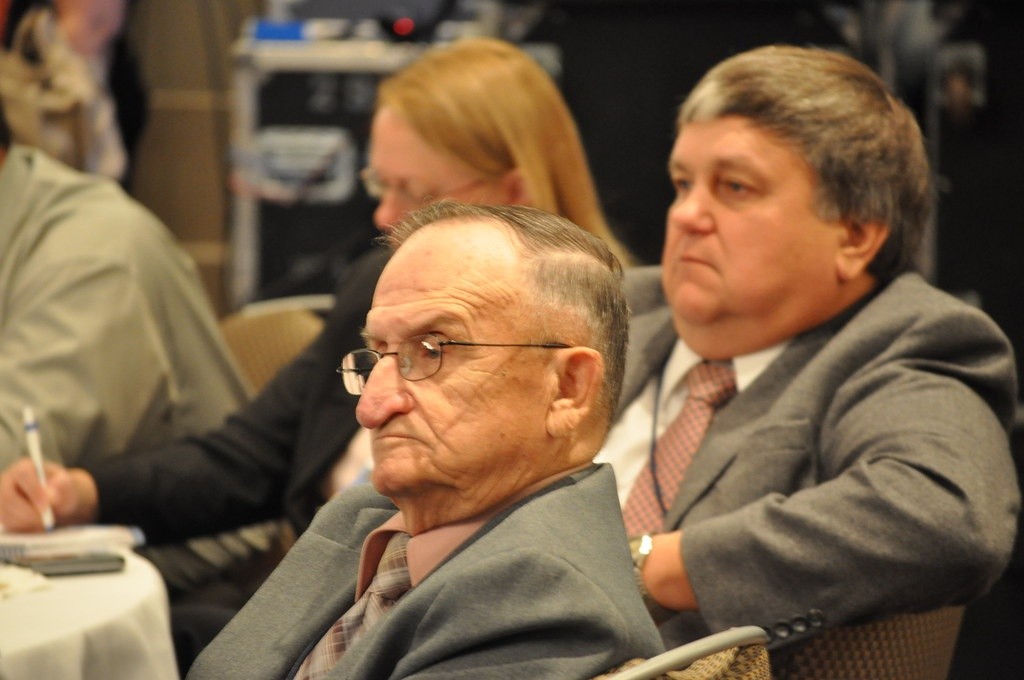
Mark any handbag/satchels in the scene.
[0,10,101,172]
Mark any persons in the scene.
[0,0,1024,680]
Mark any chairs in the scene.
[592,585,968,680]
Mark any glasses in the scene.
[359,165,488,208]
[336,333,573,395]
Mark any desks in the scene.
[0,529,185,679]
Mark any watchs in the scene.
[628,531,681,626]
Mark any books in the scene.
[0,523,131,575]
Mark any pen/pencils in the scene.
[24,405,55,532]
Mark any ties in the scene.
[622,361,738,542]
[292,531,411,680]
